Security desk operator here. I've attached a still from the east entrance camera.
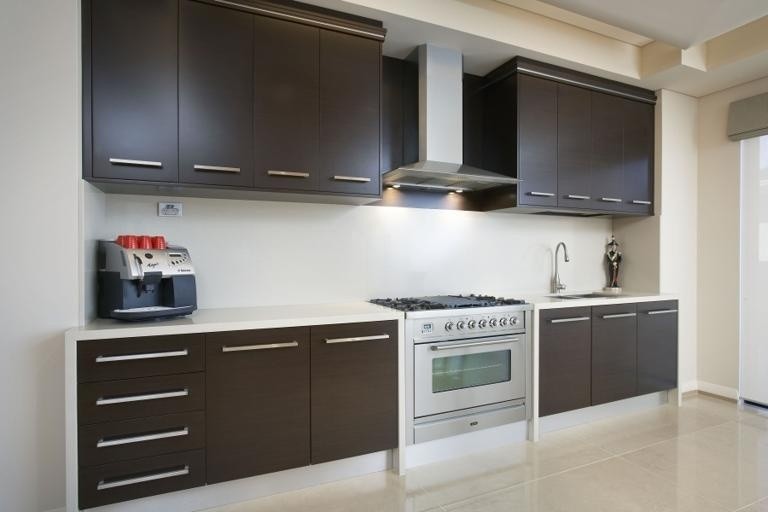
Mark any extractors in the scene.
[382,44,518,194]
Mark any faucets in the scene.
[551,241,569,293]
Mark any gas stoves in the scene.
[368,294,534,343]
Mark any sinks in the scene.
[547,295,579,300]
[571,292,615,298]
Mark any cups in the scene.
[117,235,166,250]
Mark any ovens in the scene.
[415,334,526,445]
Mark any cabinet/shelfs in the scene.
[479,55,657,217]
[64,324,206,512]
[637,300,678,395]
[310,320,398,465]
[82,0,387,204]
[539,306,591,418]
[205,325,310,484]
[591,303,636,406]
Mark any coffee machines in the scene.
[97,241,197,322]
